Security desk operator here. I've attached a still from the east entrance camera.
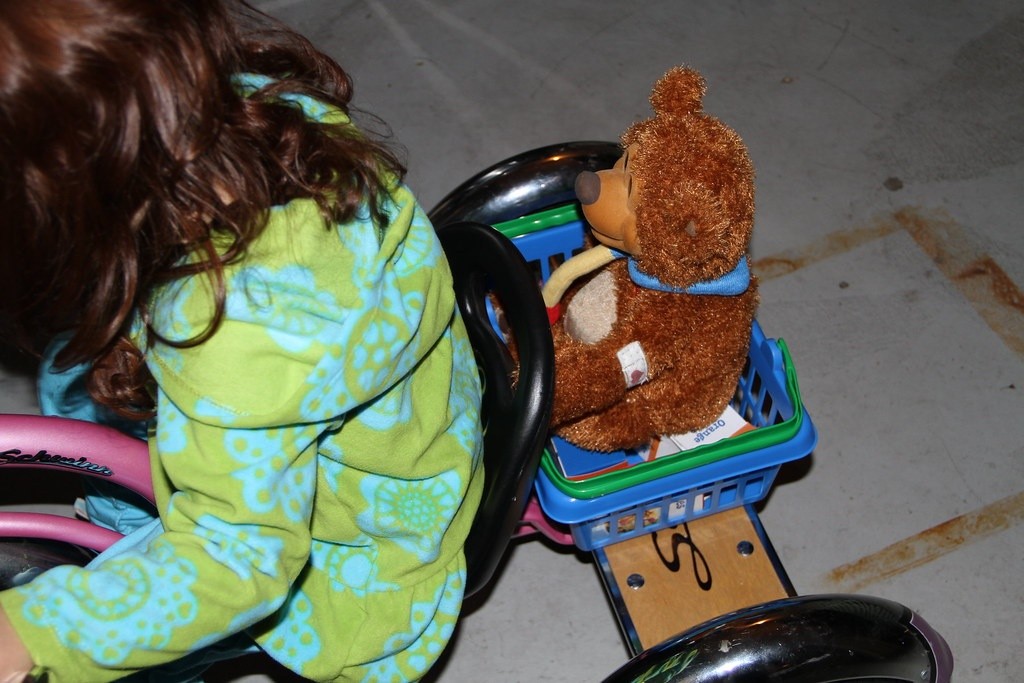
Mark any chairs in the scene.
[0,220,556,683]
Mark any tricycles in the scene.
[1,140,954,683]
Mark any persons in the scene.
[1,0,484,683]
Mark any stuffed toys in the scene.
[507,63,760,453]
